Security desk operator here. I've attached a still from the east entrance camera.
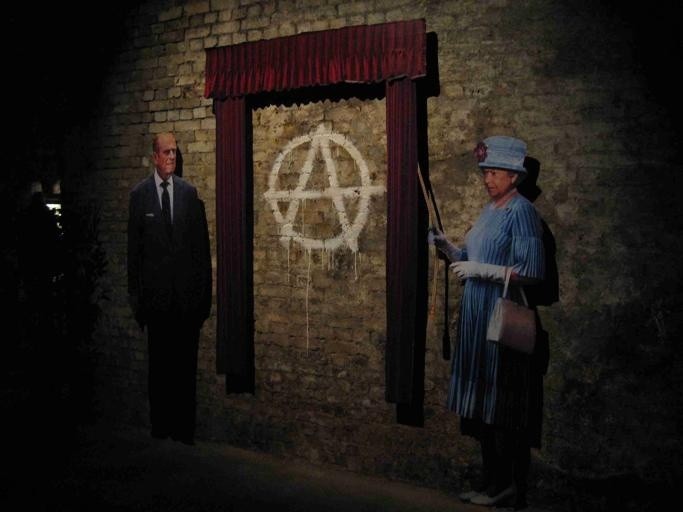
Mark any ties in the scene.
[159,180,172,232]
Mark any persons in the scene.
[428,136,542,504]
[126,133,213,447]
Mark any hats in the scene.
[478,136,528,174]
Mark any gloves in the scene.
[426,225,463,263]
[451,262,509,281]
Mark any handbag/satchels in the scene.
[486,296,536,355]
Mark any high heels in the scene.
[460,483,515,505]
